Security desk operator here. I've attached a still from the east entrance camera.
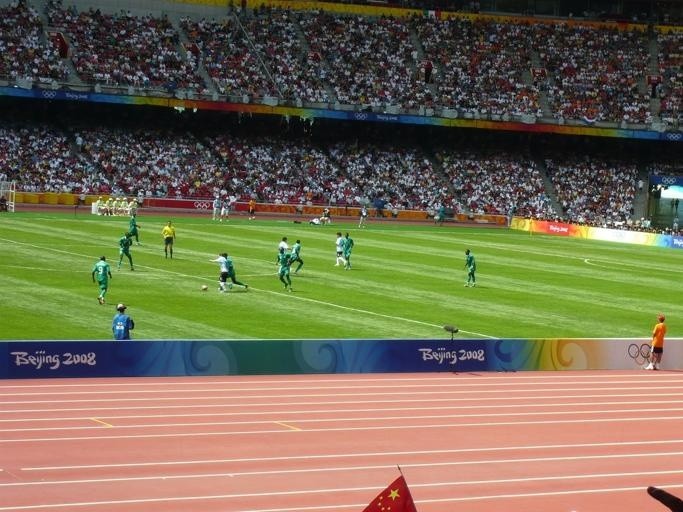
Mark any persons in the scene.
[217,253,249,291]
[111,303,134,340]
[226,0,555,230]
[287,239,304,274]
[207,253,233,293]
[2,0,260,222]
[333,232,347,267]
[275,247,293,293]
[274,236,292,266]
[462,248,477,288]
[645,486,683,512]
[160,221,175,259]
[340,233,354,270]
[644,313,667,370]
[127,211,141,246]
[529,0,683,235]
[116,232,135,271]
[91,256,112,305]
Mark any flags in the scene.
[362,475,419,512]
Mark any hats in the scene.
[657,314,666,320]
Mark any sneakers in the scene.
[97,297,105,305]
[655,365,660,370]
[644,364,655,370]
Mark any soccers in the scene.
[201,284,207,290]
